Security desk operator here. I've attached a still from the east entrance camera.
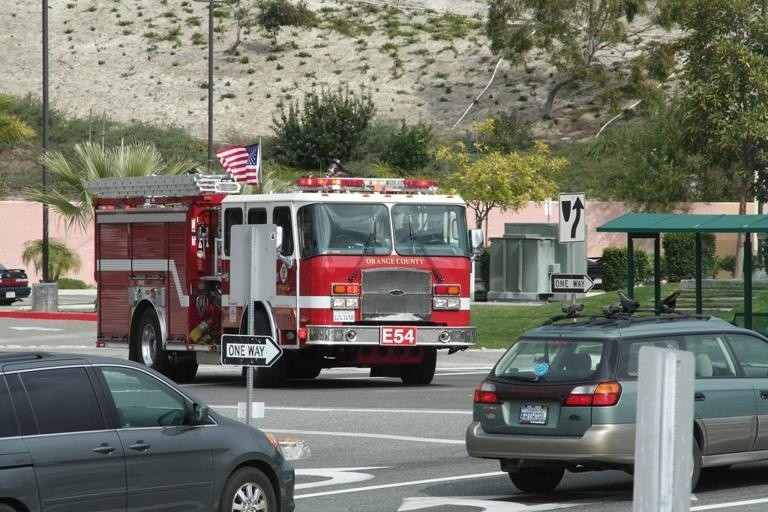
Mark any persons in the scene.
[394,212,437,245]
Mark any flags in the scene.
[215,143,265,187]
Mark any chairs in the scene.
[564,353,596,378]
[695,352,715,378]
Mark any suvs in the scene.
[463,289,766,502]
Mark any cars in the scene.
[0,344,300,512]
[0,260,33,308]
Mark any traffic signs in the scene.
[548,271,596,296]
[217,332,284,369]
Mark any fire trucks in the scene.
[79,157,480,391]
